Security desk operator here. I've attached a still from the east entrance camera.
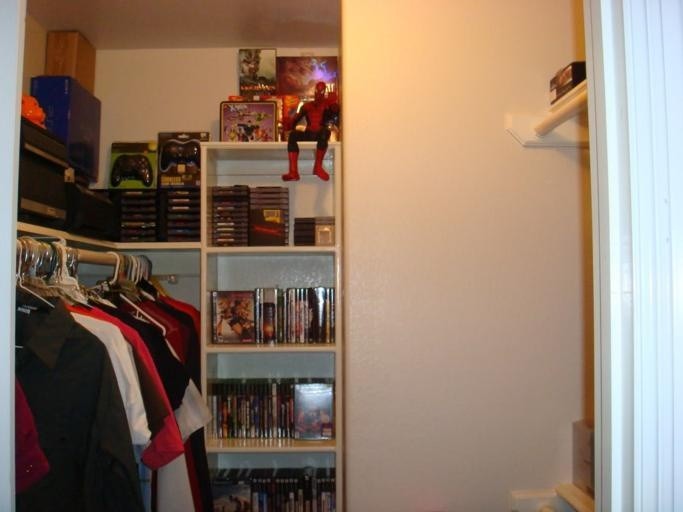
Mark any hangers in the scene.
[16,234,168,341]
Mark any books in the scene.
[208,285,335,511]
[117,179,336,249]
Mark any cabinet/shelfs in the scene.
[198,141,342,512]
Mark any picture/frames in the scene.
[220,101,278,142]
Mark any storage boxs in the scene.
[17,75,119,243]
